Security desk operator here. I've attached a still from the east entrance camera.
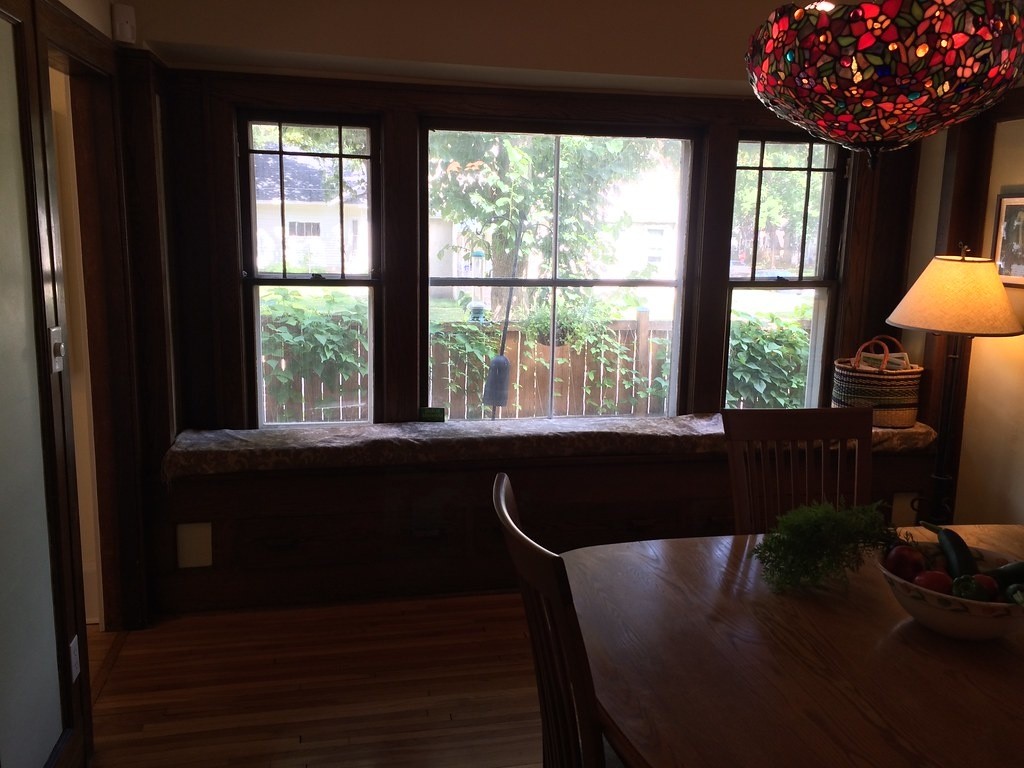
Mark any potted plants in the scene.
[744,497,915,596]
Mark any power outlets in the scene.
[70,634,81,683]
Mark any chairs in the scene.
[492,471,606,768]
[717,404,874,537]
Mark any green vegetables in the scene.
[743,498,938,601]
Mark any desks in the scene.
[560,526,1024,768]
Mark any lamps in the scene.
[744,0,1024,154]
[884,242,1024,524]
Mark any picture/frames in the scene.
[989,193,1024,288]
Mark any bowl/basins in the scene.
[871,540,1024,639]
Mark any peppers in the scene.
[953,573,989,602]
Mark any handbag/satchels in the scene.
[831,335,924,428]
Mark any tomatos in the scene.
[886,545,999,604]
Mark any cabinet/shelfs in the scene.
[156,451,908,610]
